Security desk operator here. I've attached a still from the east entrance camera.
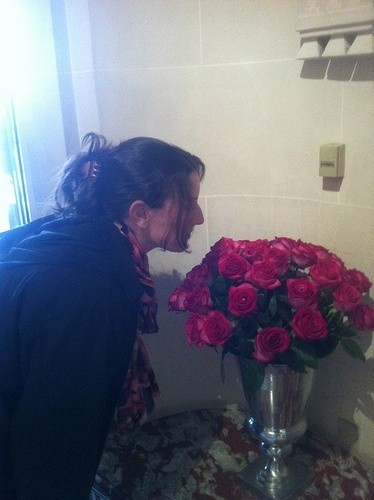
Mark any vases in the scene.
[234,357,319,500]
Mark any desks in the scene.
[90,404,374,500]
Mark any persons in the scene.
[0,131,206,499]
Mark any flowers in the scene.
[167,237,374,399]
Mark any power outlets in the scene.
[336,415,359,446]
